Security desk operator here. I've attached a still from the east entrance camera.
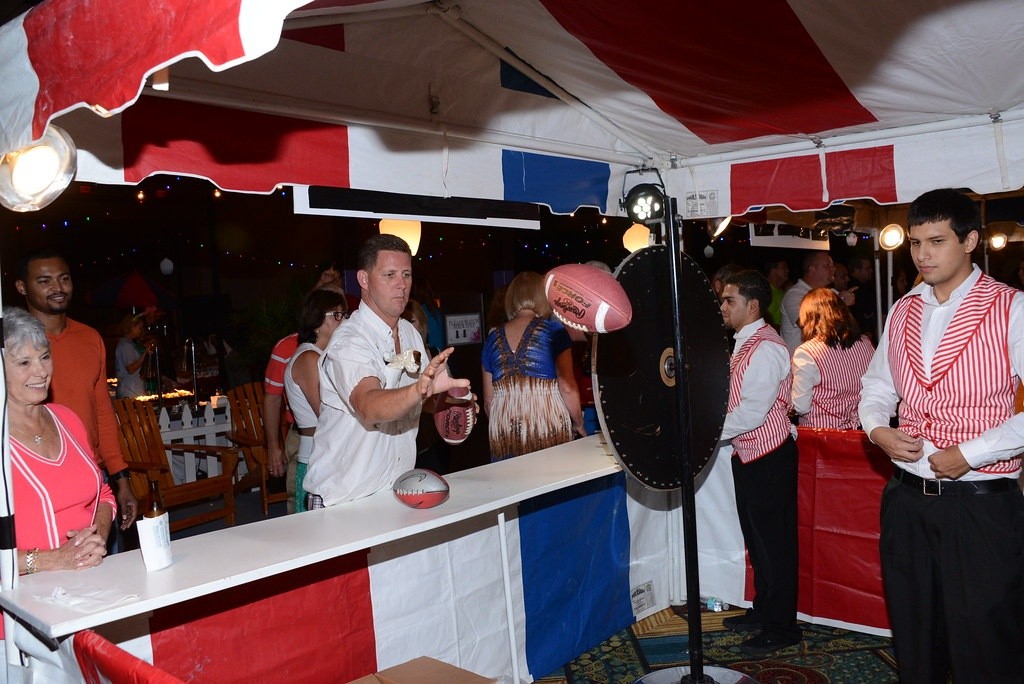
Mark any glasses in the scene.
[325,310,350,321]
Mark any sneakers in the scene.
[723,614,769,631]
[739,632,793,652]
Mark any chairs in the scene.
[227,383,298,515]
[111,397,240,537]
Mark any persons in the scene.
[15,247,138,555]
[115,314,176,399]
[482,271,586,464]
[264,263,355,515]
[766,251,876,356]
[303,234,480,510]
[3,307,117,576]
[856,189,1024,684]
[719,270,799,652]
[790,287,876,431]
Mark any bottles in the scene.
[143,480,168,571]
[700,597,729,612]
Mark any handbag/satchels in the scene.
[144,348,156,391]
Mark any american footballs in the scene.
[392,468,450,510]
[434,385,477,446]
[543,263,633,335]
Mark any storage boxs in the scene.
[343,657,498,684]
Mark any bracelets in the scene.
[112,470,128,480]
[26,548,38,575]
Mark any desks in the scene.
[1,432,632,684]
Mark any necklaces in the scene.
[33,434,42,445]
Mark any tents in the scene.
[0,0,1024,219]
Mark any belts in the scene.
[893,465,1019,496]
